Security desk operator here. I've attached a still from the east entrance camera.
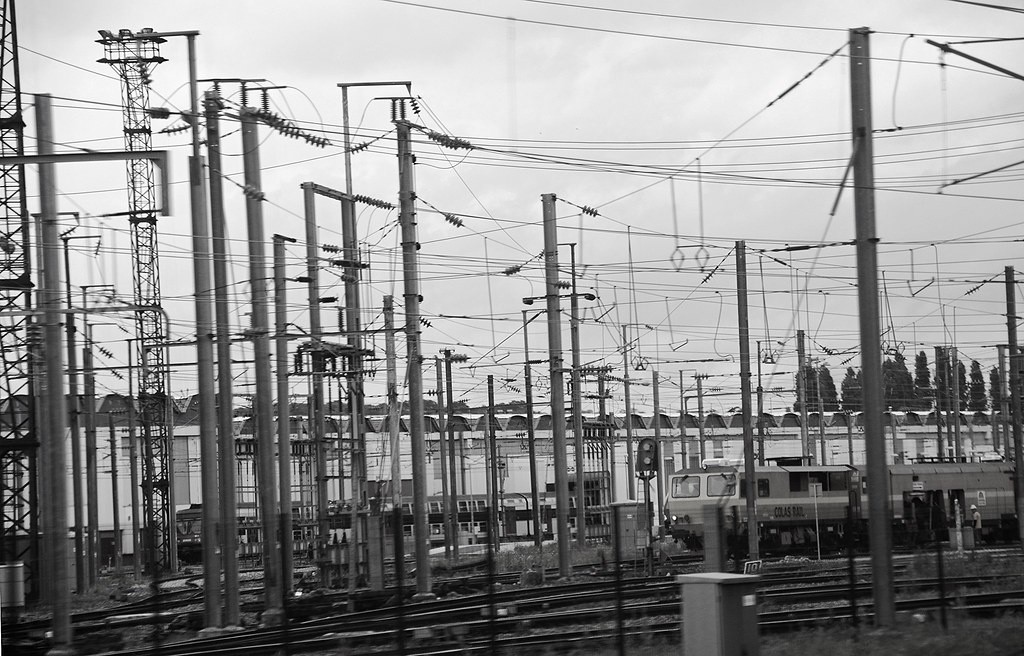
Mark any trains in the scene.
[664,458,1018,560]
